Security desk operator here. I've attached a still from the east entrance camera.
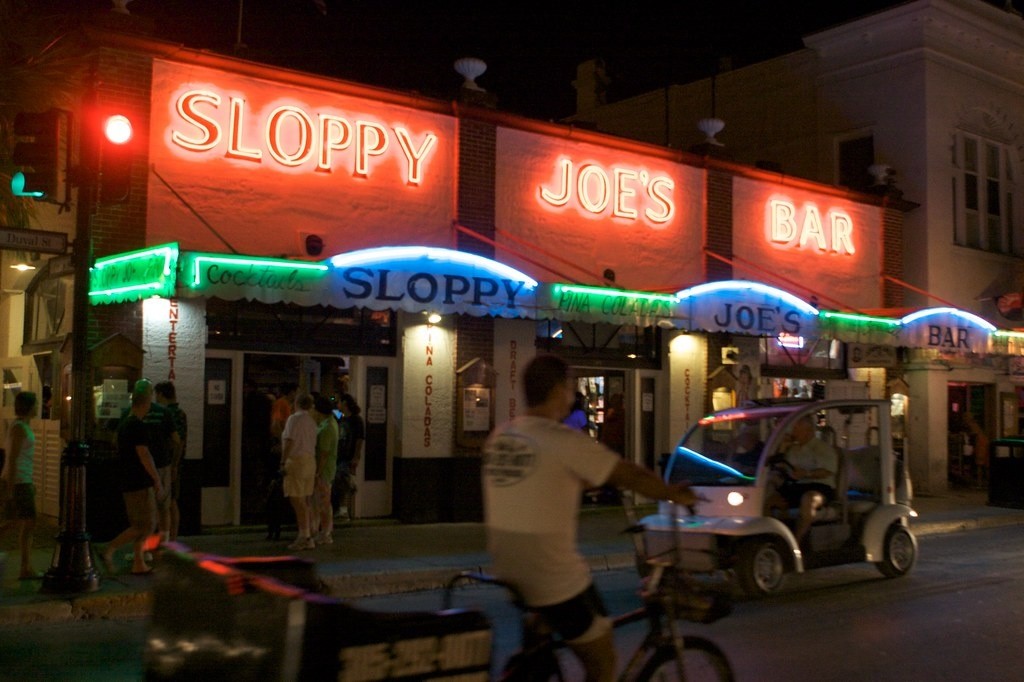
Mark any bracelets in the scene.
[280,462,284,465]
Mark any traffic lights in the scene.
[100,102,142,204]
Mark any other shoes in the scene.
[265,533,273,541]
[274,533,281,541]
[975,483,987,490]
[287,537,314,550]
[315,532,333,545]
[125,552,153,561]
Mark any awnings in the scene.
[88,241,1024,358]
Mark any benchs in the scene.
[840,446,883,542]
[766,495,849,555]
[147,541,495,681]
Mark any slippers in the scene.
[99,552,115,576]
[18,569,46,580]
[131,566,161,575]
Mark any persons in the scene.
[772,414,838,545]
[481,354,698,682]
[271,383,300,453]
[308,391,339,543]
[95,378,188,577]
[600,394,626,506]
[0,391,40,579]
[972,422,989,490]
[279,392,316,550]
[564,391,596,431]
[335,394,364,522]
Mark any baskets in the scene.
[644,547,740,623]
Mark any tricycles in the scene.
[140,488,740,681]
